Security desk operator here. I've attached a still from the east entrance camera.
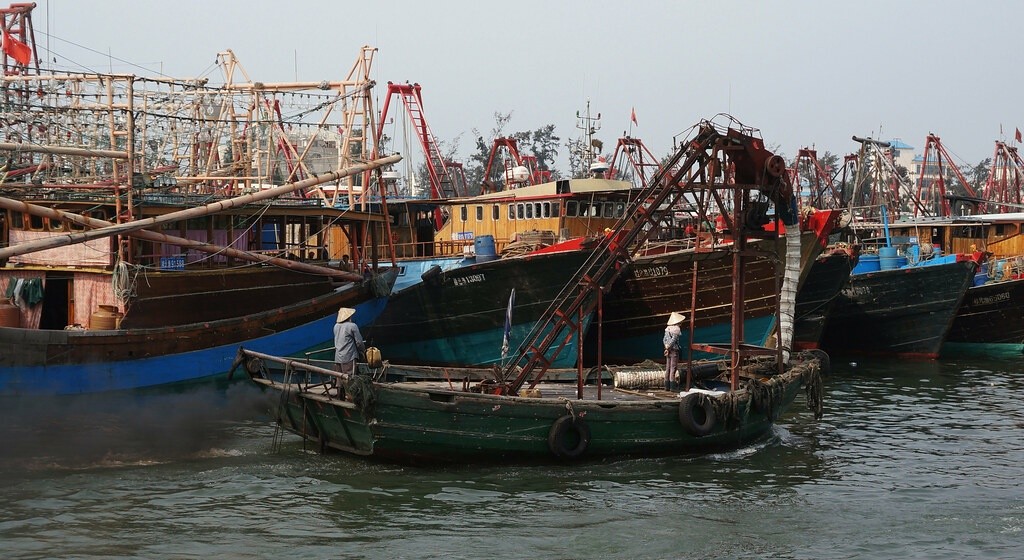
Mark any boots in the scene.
[669,381,678,392]
[664,380,670,391]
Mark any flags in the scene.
[1015,127,1022,143]
[0,28,32,68]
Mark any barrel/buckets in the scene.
[474,236,496,263]
[366,346,381,368]
[879,247,896,270]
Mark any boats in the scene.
[0,1,1024,413]
[223,113,821,461]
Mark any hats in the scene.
[666,311,686,325]
[336,307,356,322]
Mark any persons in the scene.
[333,307,367,404]
[338,254,353,272]
[663,312,686,392]
